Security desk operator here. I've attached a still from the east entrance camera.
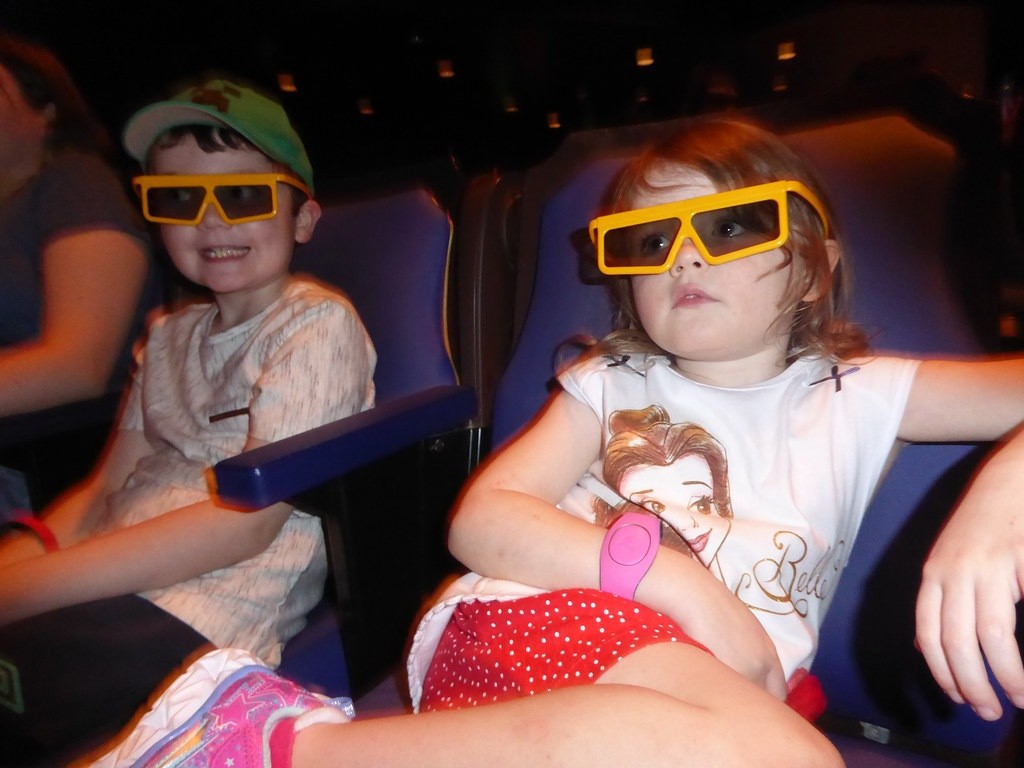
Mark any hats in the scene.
[124,80,316,190]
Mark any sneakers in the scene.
[78,644,356,767]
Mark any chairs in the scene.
[0,77,1024,768]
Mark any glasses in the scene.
[132,173,313,227]
[587,178,828,276]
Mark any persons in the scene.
[0,84,374,768]
[55,111,1024,768]
[0,29,152,542]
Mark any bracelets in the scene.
[595,510,663,609]
